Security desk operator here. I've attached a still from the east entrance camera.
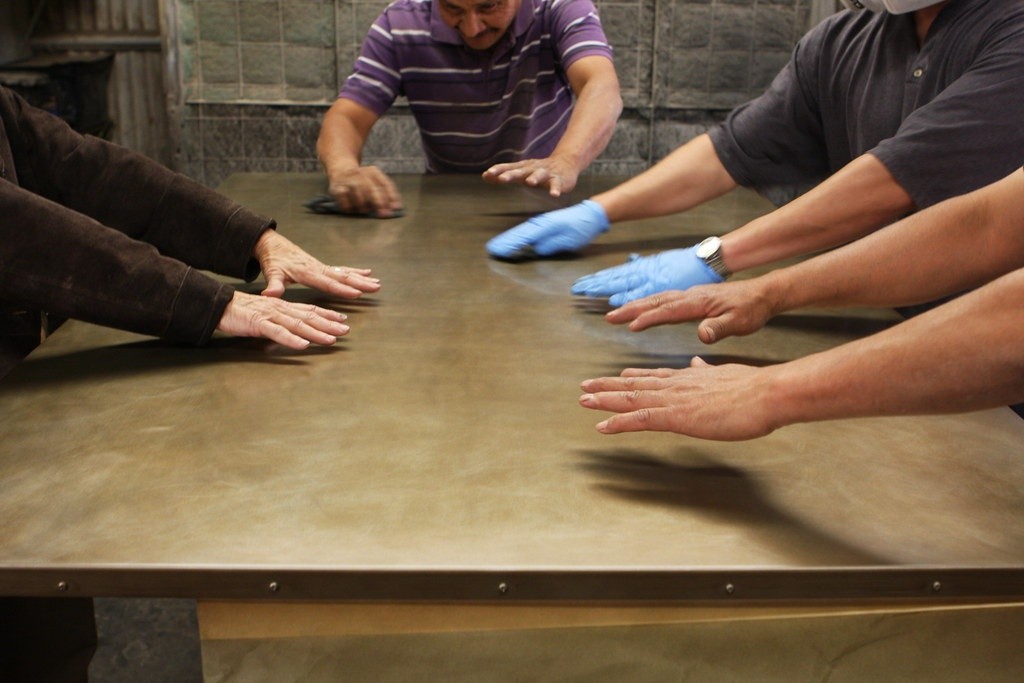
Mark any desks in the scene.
[0,169,1024,683]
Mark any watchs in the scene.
[695,236,733,278]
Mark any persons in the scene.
[579,165,1024,441]
[317,0,623,216]
[0,81,381,374]
[486,0,1024,306]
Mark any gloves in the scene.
[570,245,725,307]
[485,199,611,261]
